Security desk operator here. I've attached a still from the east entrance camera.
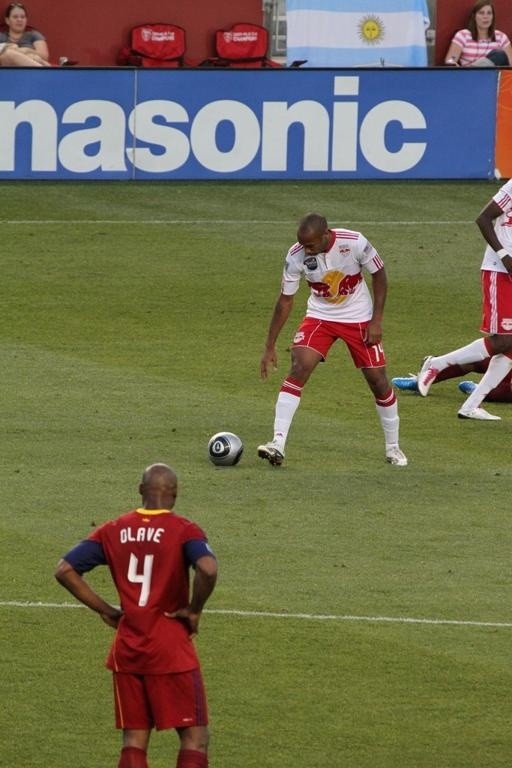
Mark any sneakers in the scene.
[458,380,478,395]
[416,355,440,396]
[386,447,408,466]
[255,441,285,467]
[391,375,420,394]
[456,406,502,422]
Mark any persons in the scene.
[392,177,512,421]
[256,213,410,468]
[55,462,218,768]
[0,3,50,66]
[445,0,512,67]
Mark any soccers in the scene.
[207,432,243,465]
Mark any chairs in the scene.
[117,19,197,67]
[1,22,62,67]
[201,22,284,69]
